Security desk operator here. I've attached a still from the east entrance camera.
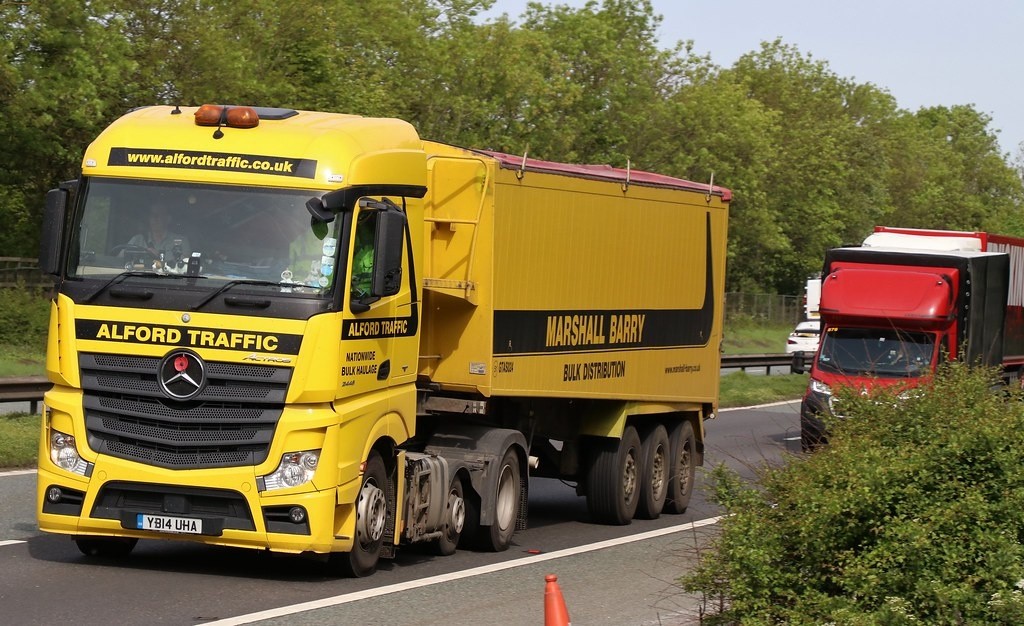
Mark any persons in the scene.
[287,212,375,298]
[116,203,191,260]
[877,339,916,367]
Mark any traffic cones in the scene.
[540,572,576,625]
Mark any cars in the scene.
[786,318,822,356]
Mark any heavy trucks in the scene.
[35,107,731,580]
[790,227,1022,457]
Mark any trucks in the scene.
[802,277,822,321]
